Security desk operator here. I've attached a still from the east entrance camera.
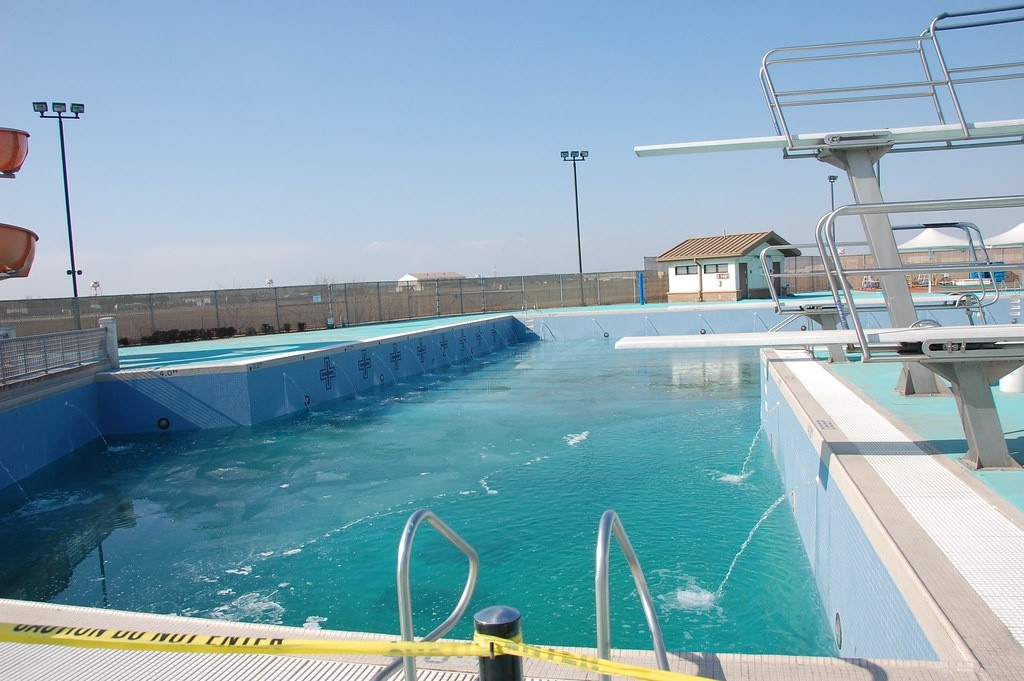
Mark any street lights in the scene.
[561,150,589,307]
[828,174,839,245]
[33,100,86,332]
